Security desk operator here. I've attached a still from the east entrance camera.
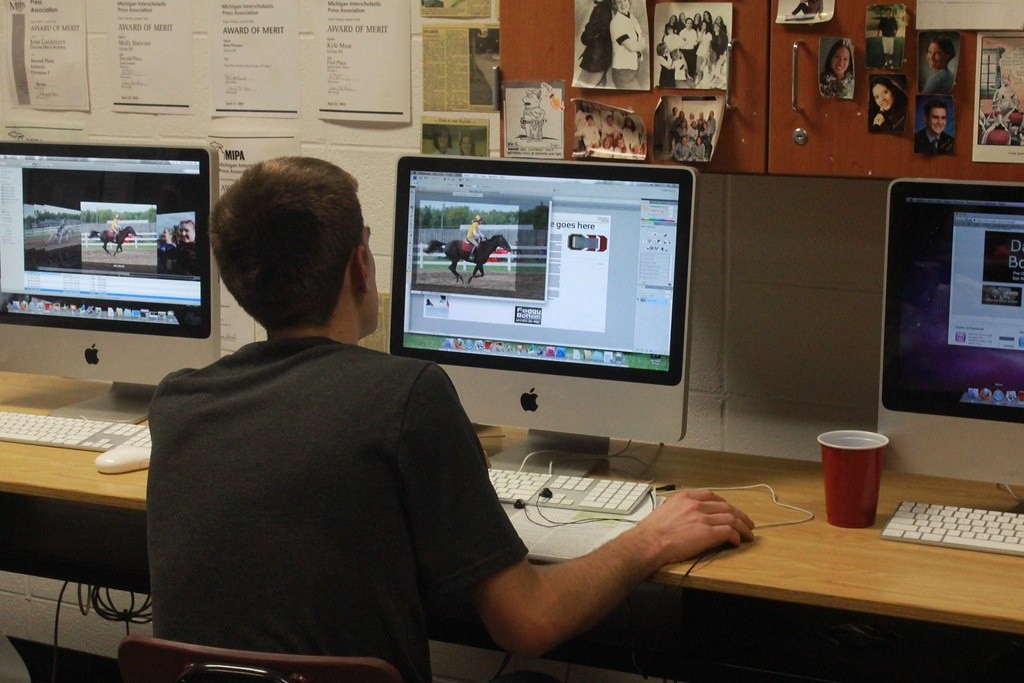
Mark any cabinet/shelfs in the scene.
[493,0,1024,185]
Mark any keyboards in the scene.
[488,468,653,515]
[0,412,152,453]
[879,501,1024,557]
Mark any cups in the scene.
[817,430,889,529]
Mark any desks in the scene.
[0,371,156,511]
[476,427,1024,683]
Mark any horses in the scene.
[44,225,75,246]
[87,226,137,256]
[422,234,512,285]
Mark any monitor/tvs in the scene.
[386,154,698,474]
[0,140,220,424]
[877,177,1024,486]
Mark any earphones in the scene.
[514,499,525,509]
[539,488,552,497]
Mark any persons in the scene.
[575,0,728,161]
[465,212,486,265]
[149,154,758,683]
[58,217,66,234]
[110,213,121,243]
[428,124,480,153]
[788,0,956,154]
[157,219,197,275]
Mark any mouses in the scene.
[95,446,152,474]
